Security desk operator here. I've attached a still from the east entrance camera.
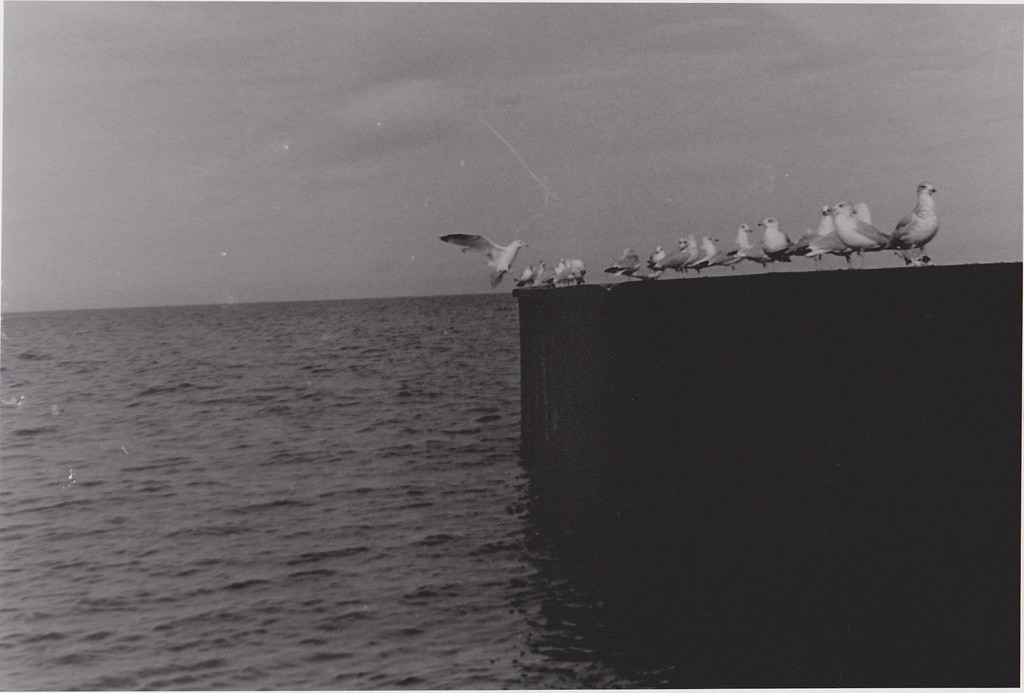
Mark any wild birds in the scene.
[438,184,939,289]
